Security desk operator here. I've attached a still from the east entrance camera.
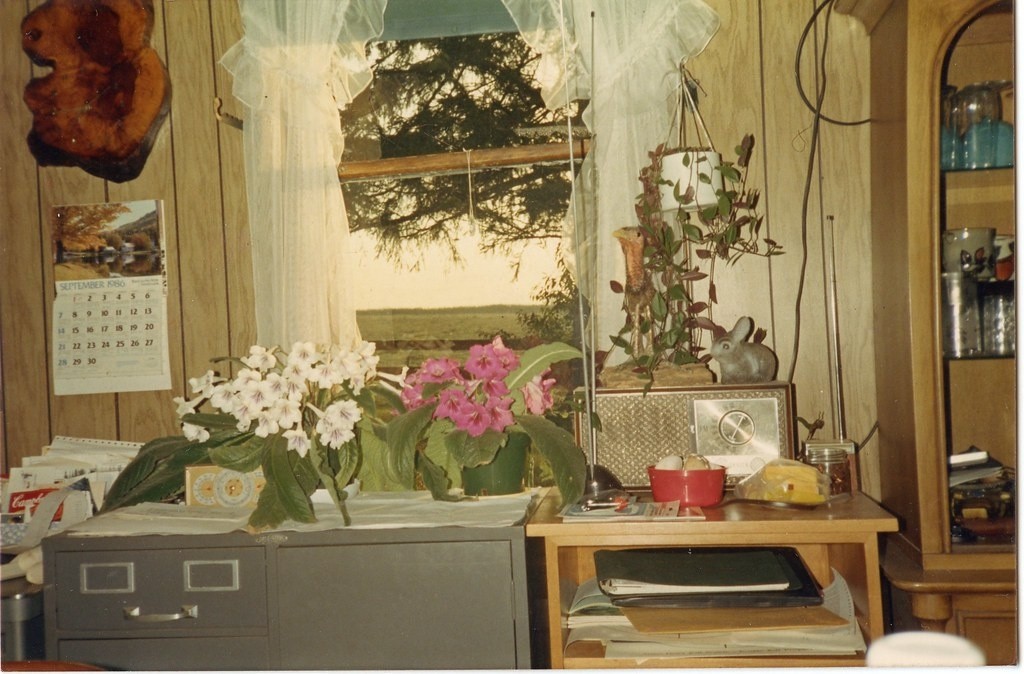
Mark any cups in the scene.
[942,227,1014,282]
[940,81,1013,169]
[940,271,981,358]
[978,279,1016,356]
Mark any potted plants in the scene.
[609,133,786,397]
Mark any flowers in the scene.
[94,339,408,534]
[386,333,590,506]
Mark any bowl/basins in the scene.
[646,464,727,507]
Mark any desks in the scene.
[525,485,899,669]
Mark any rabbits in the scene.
[711,316,778,384]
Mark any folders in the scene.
[594,548,823,608]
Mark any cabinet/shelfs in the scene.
[40,486,553,670]
[833,0,1016,667]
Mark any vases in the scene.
[463,432,531,497]
[317,449,355,489]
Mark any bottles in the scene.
[807,448,851,500]
